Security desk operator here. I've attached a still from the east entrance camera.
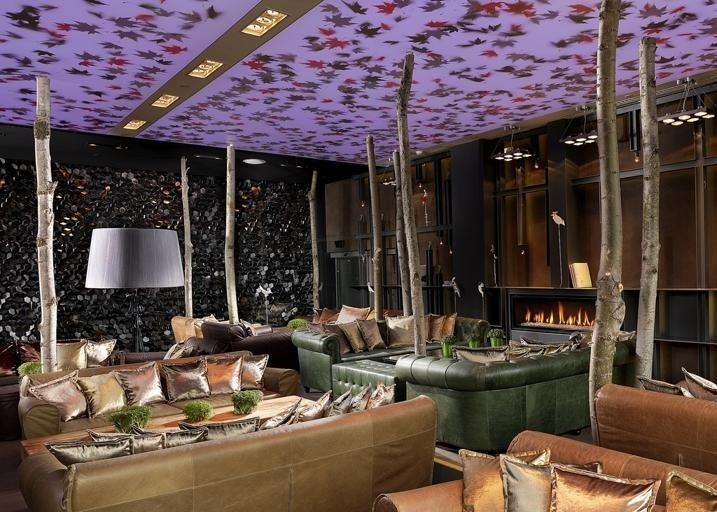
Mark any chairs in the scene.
[201,322,299,375]
[170,314,262,343]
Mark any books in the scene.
[569,263,593,288]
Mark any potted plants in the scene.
[439,335,457,358]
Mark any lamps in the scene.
[654,76,717,126]
[490,124,535,163]
[379,157,399,187]
[85,228,183,352]
[558,105,599,147]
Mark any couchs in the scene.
[395,339,636,453]
[372,430,717,512]
[291,313,491,402]
[593,379,717,475]
[16,394,436,512]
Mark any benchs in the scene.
[331,359,406,403]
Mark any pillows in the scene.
[549,459,661,511]
[498,454,603,512]
[636,375,696,400]
[681,366,717,402]
[458,449,550,512]
[240,318,257,336]
[664,469,717,512]
[452,329,636,363]
[311,304,457,355]
